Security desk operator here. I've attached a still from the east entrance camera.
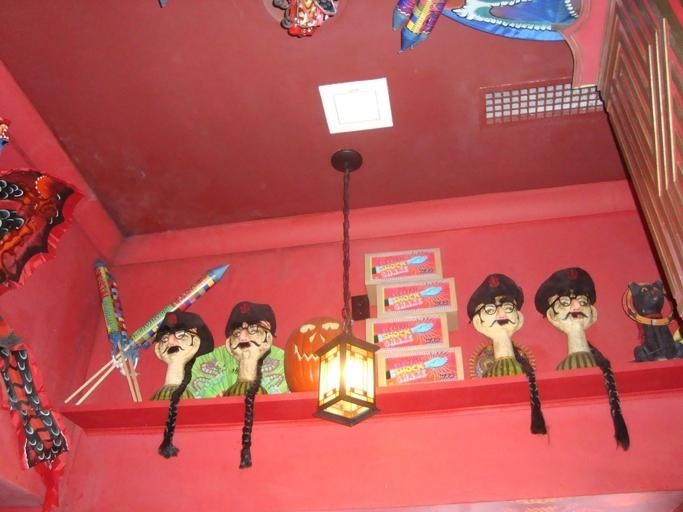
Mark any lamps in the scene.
[310,146,384,427]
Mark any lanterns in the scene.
[282,316,346,394]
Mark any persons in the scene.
[147,311,213,460]
[467,272,547,436]
[216,300,275,469]
[535,266,629,450]
